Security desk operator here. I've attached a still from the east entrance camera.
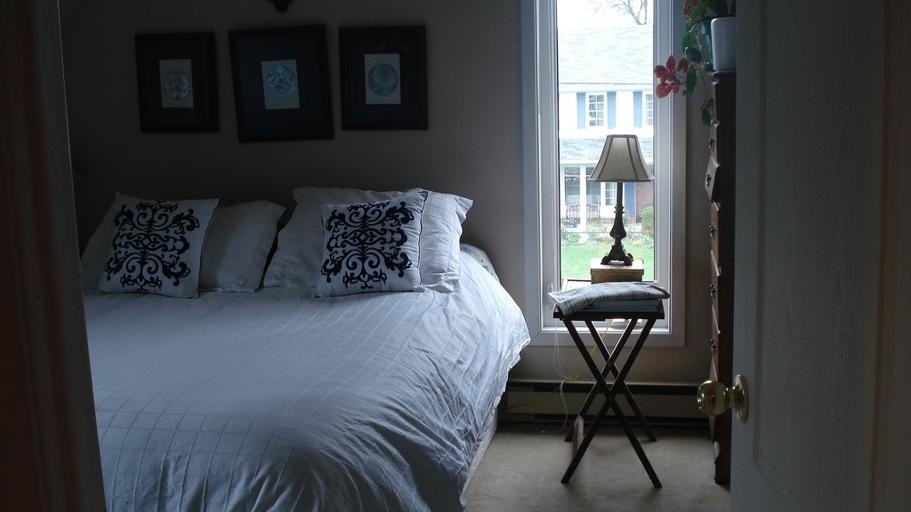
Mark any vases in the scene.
[711,17,736,71]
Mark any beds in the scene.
[79,242,531,512]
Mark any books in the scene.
[574,299,659,312]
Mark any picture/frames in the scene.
[134,22,429,144]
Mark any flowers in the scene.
[653,0,736,127]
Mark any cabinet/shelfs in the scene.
[704,71,737,485]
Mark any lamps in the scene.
[587,134,655,266]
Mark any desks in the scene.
[553,278,664,489]
